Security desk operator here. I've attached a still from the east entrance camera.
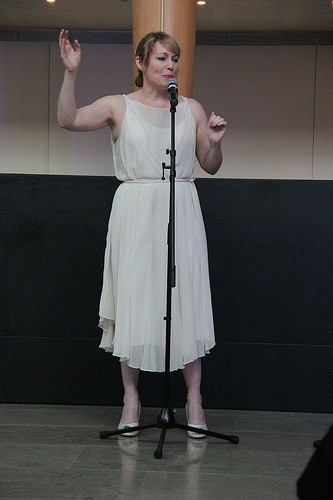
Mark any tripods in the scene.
[99,104,240,459]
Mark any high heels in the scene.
[185,403,208,438]
[118,401,141,436]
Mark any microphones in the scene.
[167,78,178,106]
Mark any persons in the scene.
[56,26,229,439]
